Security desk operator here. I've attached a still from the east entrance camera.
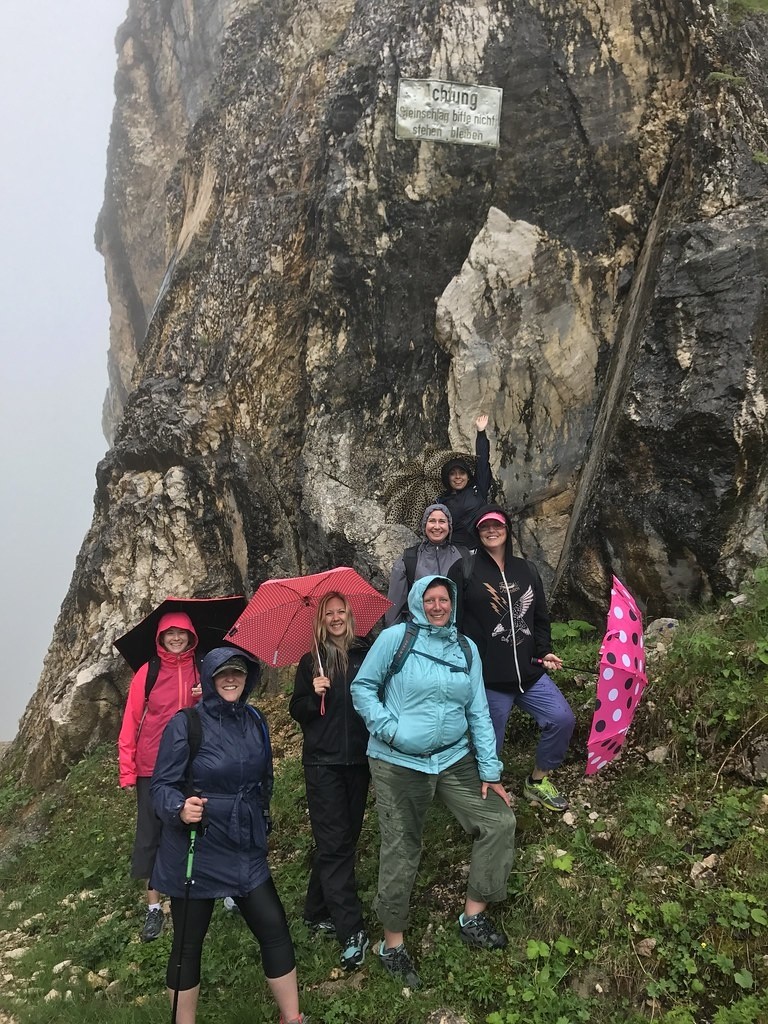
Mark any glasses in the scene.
[478,522,510,532]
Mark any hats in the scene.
[476,511,506,527]
[211,656,249,676]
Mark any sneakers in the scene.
[378,941,418,983]
[458,913,510,950]
[224,896,237,911]
[340,930,368,968]
[522,775,569,811]
[304,917,338,932]
[143,907,164,940]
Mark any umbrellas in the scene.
[111,595,247,673]
[224,566,398,696]
[382,446,484,536]
[531,572,650,780]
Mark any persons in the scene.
[445,511,575,813]
[351,575,518,975]
[442,414,506,578]
[148,648,309,1023]
[288,588,386,968]
[117,610,207,941]
[383,504,466,628]
